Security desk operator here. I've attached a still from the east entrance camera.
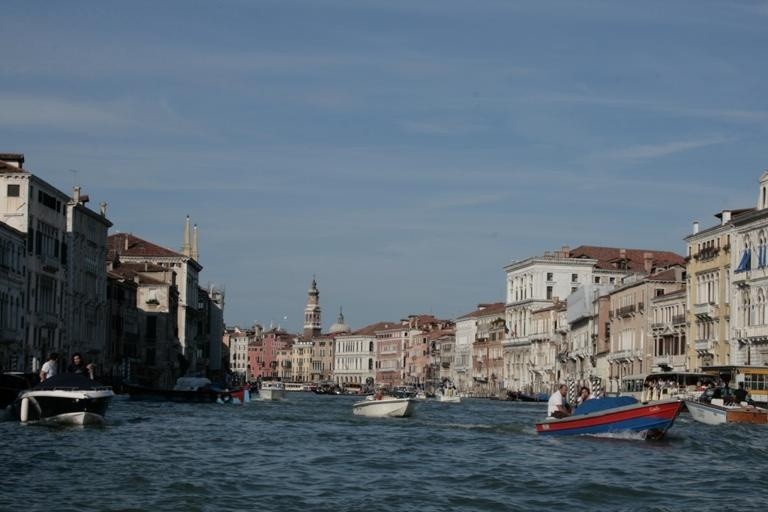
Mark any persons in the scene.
[644,376,747,405]
[68,353,92,376]
[547,385,571,417]
[39,352,59,383]
[366,388,384,400]
[576,387,595,405]
[434,380,458,399]
[224,369,238,386]
[565,402,572,413]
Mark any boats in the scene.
[348,393,419,422]
[14,375,121,426]
[486,385,551,407]
[281,378,460,405]
[533,390,684,450]
[616,352,768,425]
[119,376,253,410]
[256,376,288,404]
